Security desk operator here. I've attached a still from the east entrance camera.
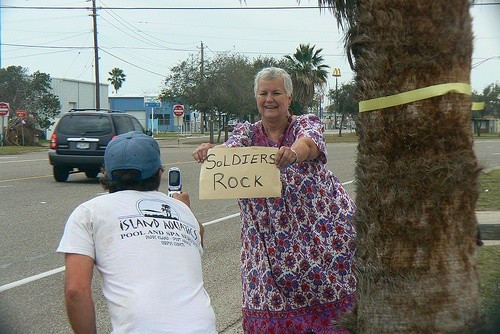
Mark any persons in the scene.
[55,131,219,334]
[192,67,357,334]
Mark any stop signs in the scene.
[172,101,185,118]
[1,100,10,118]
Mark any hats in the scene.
[105,131,161,180]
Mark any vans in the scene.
[49,109,153,188]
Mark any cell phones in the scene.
[168,167,183,197]
